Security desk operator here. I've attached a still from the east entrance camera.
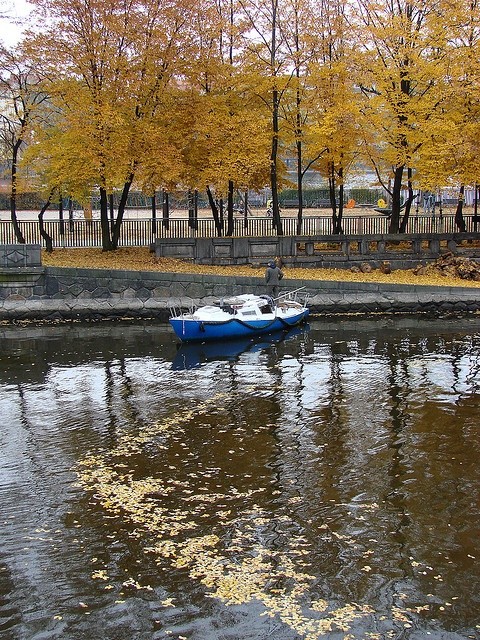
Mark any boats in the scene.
[168,286,310,343]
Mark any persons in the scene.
[422,190,431,213]
[265,259,284,307]
